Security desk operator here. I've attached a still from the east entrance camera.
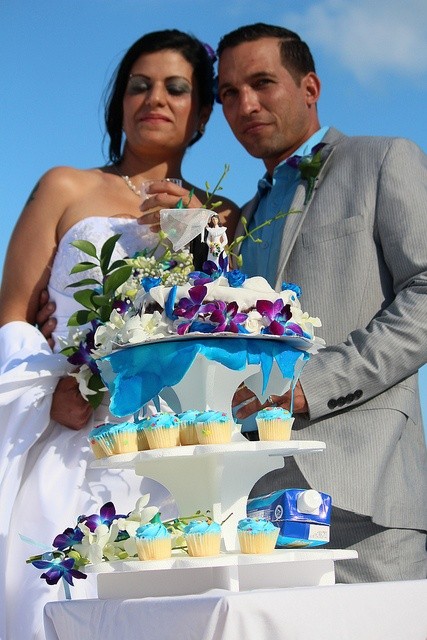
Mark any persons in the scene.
[160,208,230,274]
[0,27,242,640]
[36,22,427,584]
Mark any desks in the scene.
[41,575,426,639]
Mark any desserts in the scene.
[88,409,230,458]
[133,523,172,559]
[255,407,295,441]
[183,519,222,556]
[236,518,281,554]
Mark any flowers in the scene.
[27,493,225,598]
[63,242,324,402]
[288,140,330,205]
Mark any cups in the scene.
[142,178,183,234]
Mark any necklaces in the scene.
[114,164,170,203]
